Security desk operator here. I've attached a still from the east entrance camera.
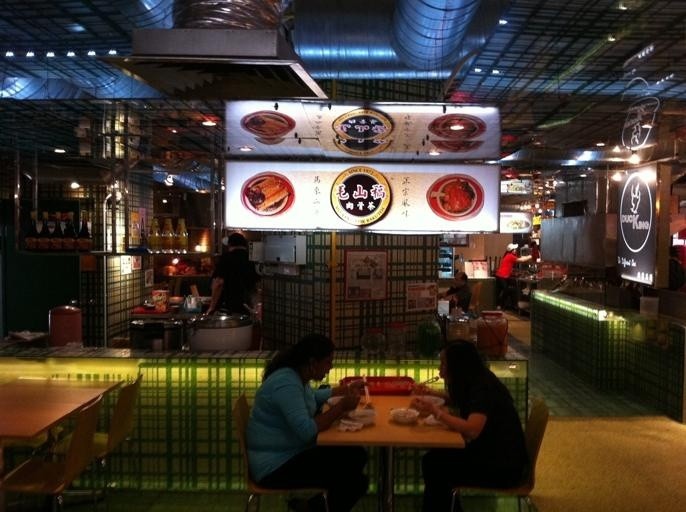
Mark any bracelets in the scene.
[435,410,443,421]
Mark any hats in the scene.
[506,244,518,252]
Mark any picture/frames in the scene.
[342,246,389,303]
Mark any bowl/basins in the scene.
[238,111,296,141]
[436,179,476,216]
[244,177,289,216]
[347,409,375,426]
[326,397,350,418]
[390,409,419,424]
[418,396,445,408]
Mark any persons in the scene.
[437,270,473,314]
[244,333,370,511]
[205,232,258,317]
[185,277,211,297]
[408,338,531,512]
[494,243,532,310]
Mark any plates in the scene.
[426,173,484,222]
[242,171,294,216]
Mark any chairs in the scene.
[449,391,553,511]
[228,387,332,512]
[53,373,145,511]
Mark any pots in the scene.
[186,308,254,352]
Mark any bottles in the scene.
[23,210,38,252]
[49,211,63,253]
[150,218,160,251]
[173,219,188,250]
[161,219,174,249]
[35,212,52,252]
[77,210,93,252]
[62,212,77,252]
